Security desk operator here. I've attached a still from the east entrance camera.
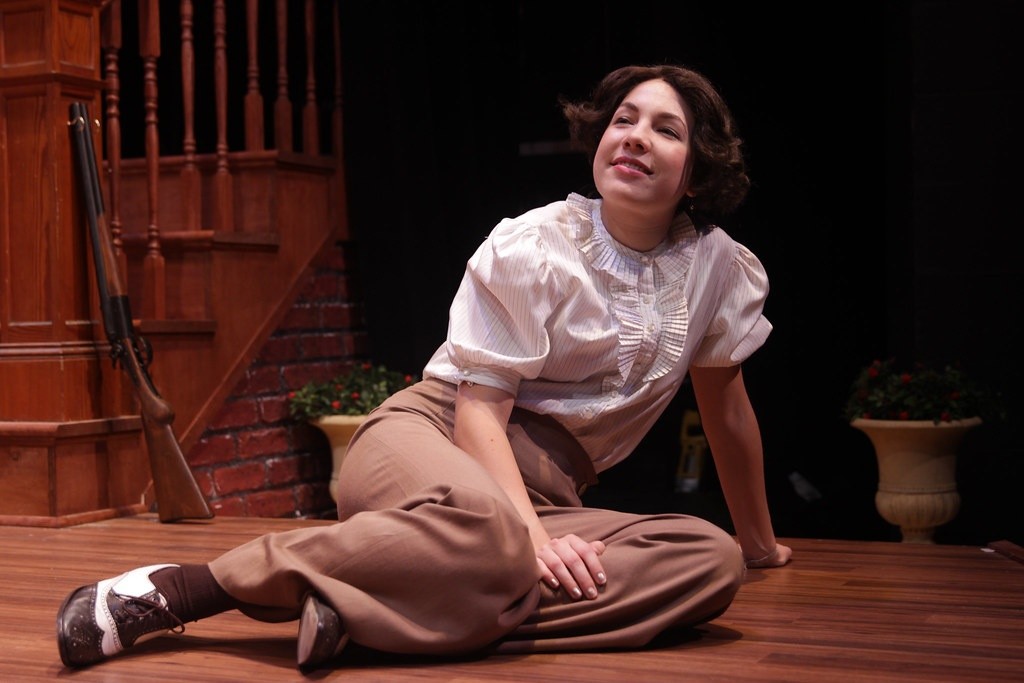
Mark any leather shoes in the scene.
[57,564,185,670]
[298,596,349,671]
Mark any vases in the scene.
[852,413,982,542]
[317,417,371,505]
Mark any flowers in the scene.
[830,358,979,428]
[284,355,410,416]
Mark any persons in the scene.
[54,66,794,678]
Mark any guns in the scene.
[69,100,215,523]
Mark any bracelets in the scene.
[744,547,778,567]
